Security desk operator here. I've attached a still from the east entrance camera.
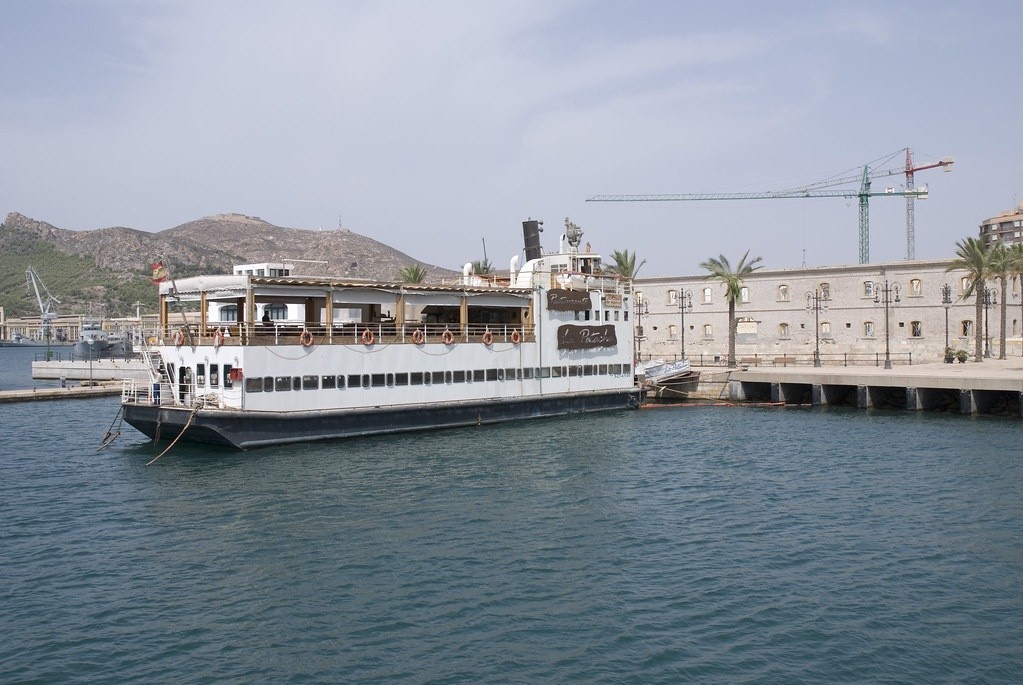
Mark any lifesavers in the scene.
[441,330,454,345]
[300,331,314,346]
[361,329,375,346]
[483,332,494,345]
[173,330,184,347]
[412,329,424,345]
[212,331,223,347]
[511,331,521,344]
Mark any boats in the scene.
[122,219,651,453]
[30,313,153,384]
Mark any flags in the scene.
[152,260,167,284]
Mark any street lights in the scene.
[805,288,829,368]
[674,288,694,359]
[939,282,952,362]
[873,279,901,371]
[982,286,998,358]
[633,296,649,365]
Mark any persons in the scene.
[179,366,186,402]
[186,367,196,392]
[152,368,163,405]
[262,311,270,322]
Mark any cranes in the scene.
[583,164,931,263]
[769,147,955,261]
